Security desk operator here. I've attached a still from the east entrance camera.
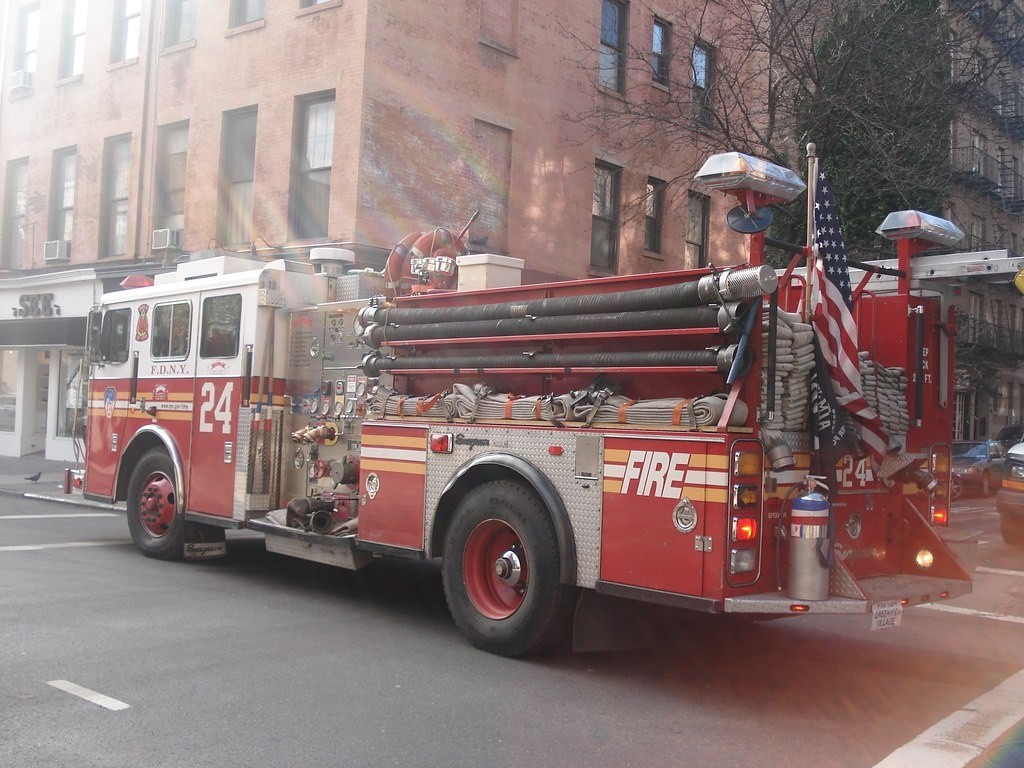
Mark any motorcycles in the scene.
[931,468,964,501]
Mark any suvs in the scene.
[994,425,1024,517]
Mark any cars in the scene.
[951,439,1008,497]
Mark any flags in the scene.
[809,157,888,475]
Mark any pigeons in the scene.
[23,471,43,484]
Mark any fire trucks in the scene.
[62,150,971,658]
